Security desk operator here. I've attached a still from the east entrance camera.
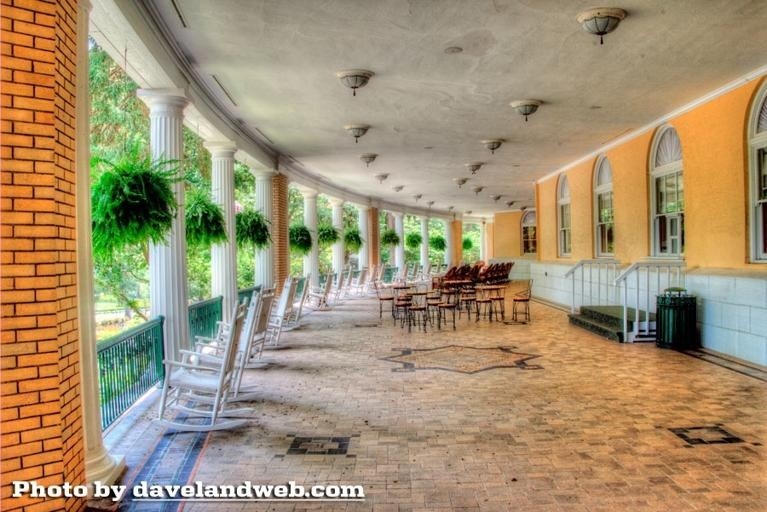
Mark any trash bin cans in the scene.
[656,296,696,348]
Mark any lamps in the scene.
[343,124,368,144]
[337,69,374,96]
[480,139,504,155]
[574,8,627,46]
[509,99,542,122]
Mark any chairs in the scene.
[152,266,369,432]
[369,261,533,333]
